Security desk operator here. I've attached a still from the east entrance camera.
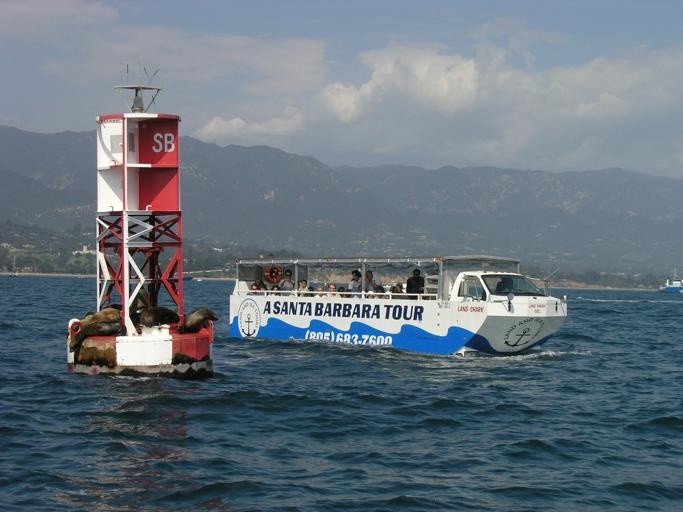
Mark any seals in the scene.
[66,284,221,353]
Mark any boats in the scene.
[658,279,680,293]
[228,255,568,357]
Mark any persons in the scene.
[270,285,280,294]
[396,283,404,293]
[250,283,260,294]
[352,284,362,298]
[278,269,295,290]
[306,285,315,297]
[336,286,347,297]
[326,282,341,298]
[345,269,361,298]
[313,285,327,298]
[371,279,385,299]
[499,278,517,296]
[298,280,310,297]
[257,284,267,290]
[364,269,385,298]
[406,268,424,299]
